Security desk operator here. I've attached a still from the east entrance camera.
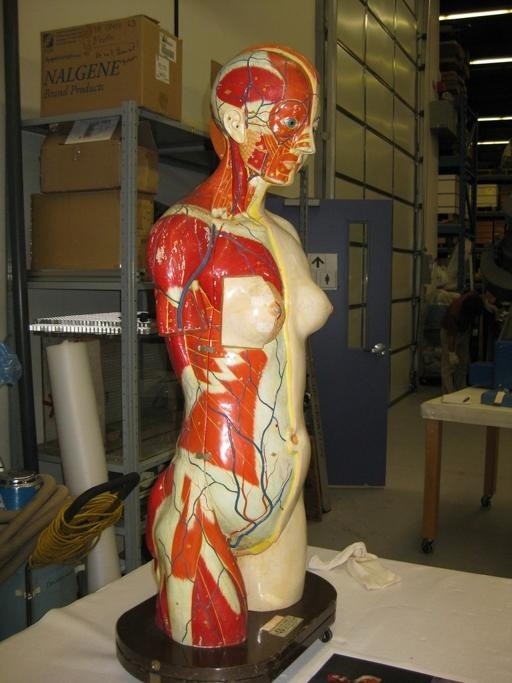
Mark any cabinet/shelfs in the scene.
[22,105,220,579]
[421,67,511,385]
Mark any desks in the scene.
[420,386,512,553]
[0,541,512,683]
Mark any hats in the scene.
[480,232,512,291]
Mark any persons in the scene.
[146,43,335,648]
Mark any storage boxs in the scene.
[40,119,160,194]
[31,191,157,281]
[41,14,183,119]
